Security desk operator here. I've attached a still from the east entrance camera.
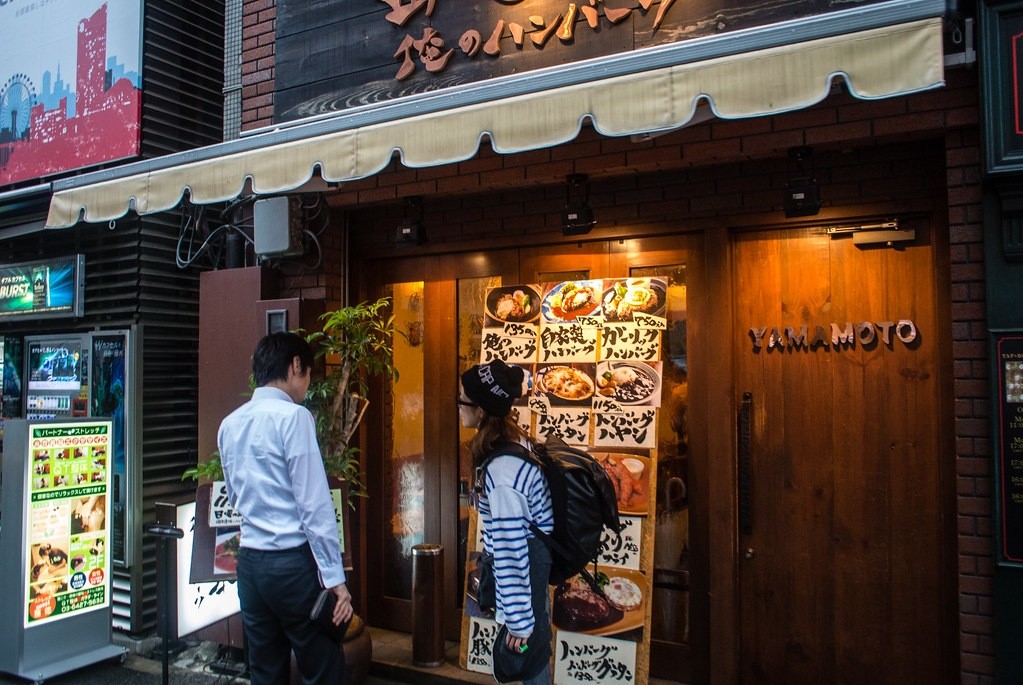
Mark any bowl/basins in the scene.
[596,361,661,405]
[485,285,541,324]
[602,278,666,320]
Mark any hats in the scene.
[461,358,524,417]
[492,618,553,683]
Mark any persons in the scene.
[456,359,556,685]
[33,543,70,582]
[75,493,107,533]
[217,331,354,685]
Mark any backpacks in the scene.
[475,434,620,590]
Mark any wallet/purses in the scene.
[310,589,353,638]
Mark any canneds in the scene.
[32,265,50,309]
[43,354,75,377]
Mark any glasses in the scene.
[456,392,478,406]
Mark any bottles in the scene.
[30,397,68,419]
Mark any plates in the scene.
[586,452,650,516]
[536,365,595,400]
[541,281,601,324]
[563,565,646,637]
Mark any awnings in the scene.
[44,2,959,234]
